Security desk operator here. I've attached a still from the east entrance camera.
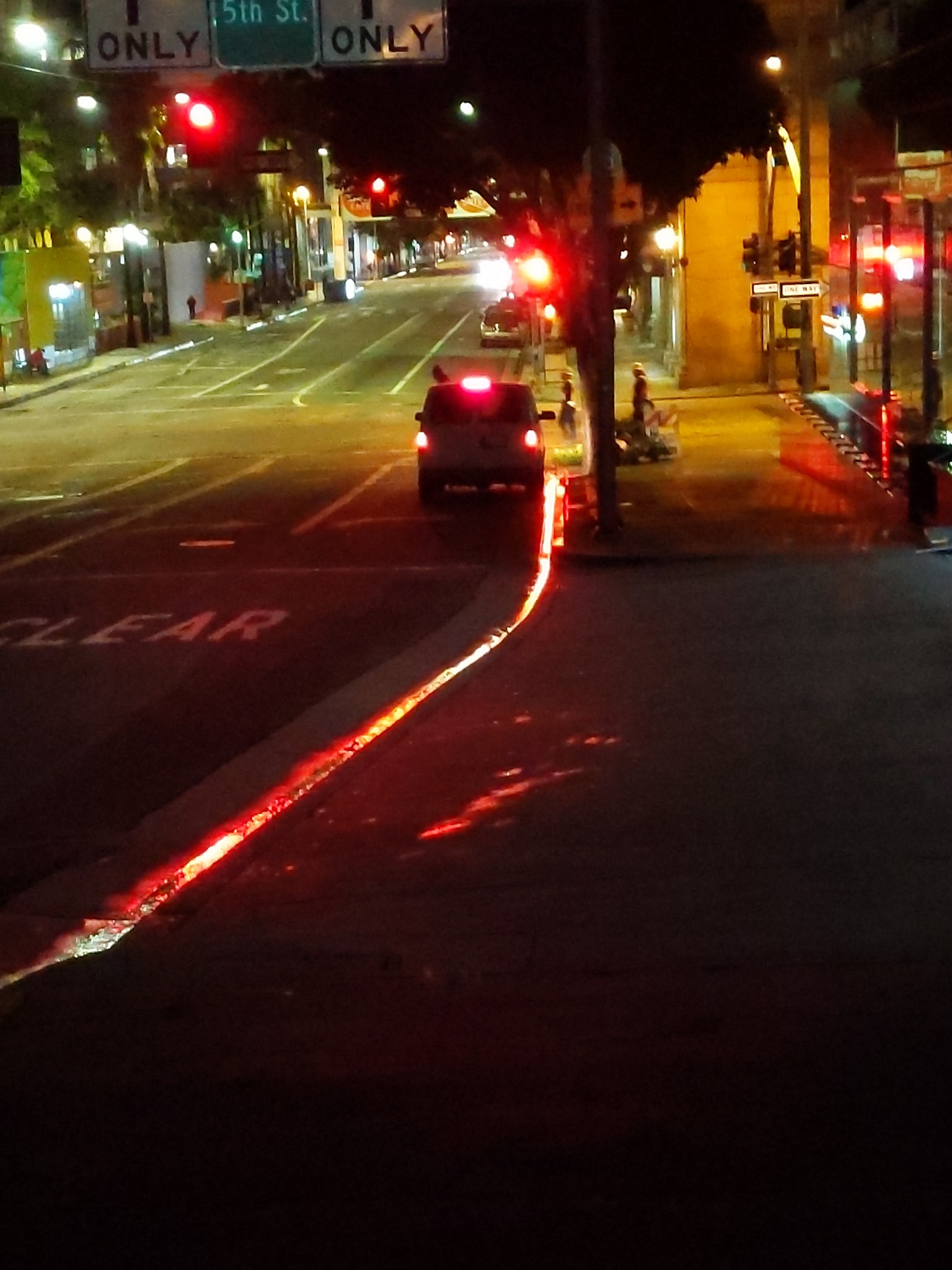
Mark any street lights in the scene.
[296,185,315,293]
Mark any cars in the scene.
[414,376,556,501]
[478,302,527,346]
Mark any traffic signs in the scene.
[318,0,448,68]
[750,281,780,297]
[778,278,829,300]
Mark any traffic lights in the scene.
[770,133,788,167]
[778,229,797,277]
[183,84,232,167]
[741,233,761,276]
[370,172,390,218]
[231,230,245,251]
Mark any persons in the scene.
[629,360,650,433]
[921,349,946,422]
[558,364,578,442]
[186,294,197,320]
[27,348,49,376]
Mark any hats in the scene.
[632,362,644,372]
[560,365,573,375]
